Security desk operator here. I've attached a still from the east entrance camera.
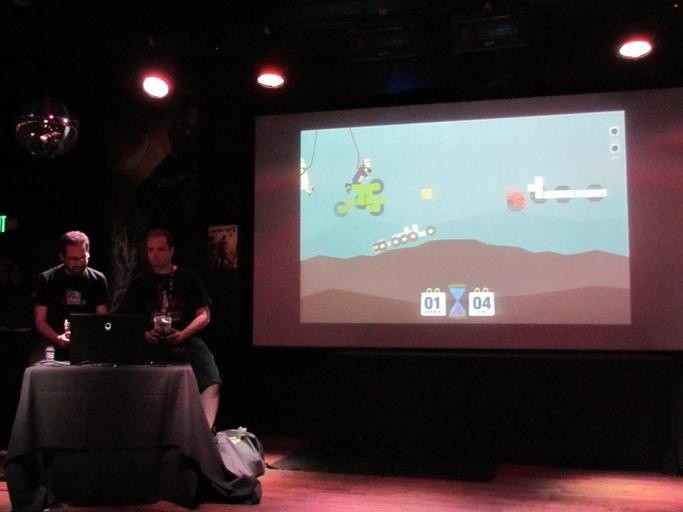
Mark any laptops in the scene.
[69,314,152,364]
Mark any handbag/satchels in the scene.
[216,430,265,479]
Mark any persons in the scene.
[116,227,223,432]
[33,229,112,361]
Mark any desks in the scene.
[21,362,195,504]
